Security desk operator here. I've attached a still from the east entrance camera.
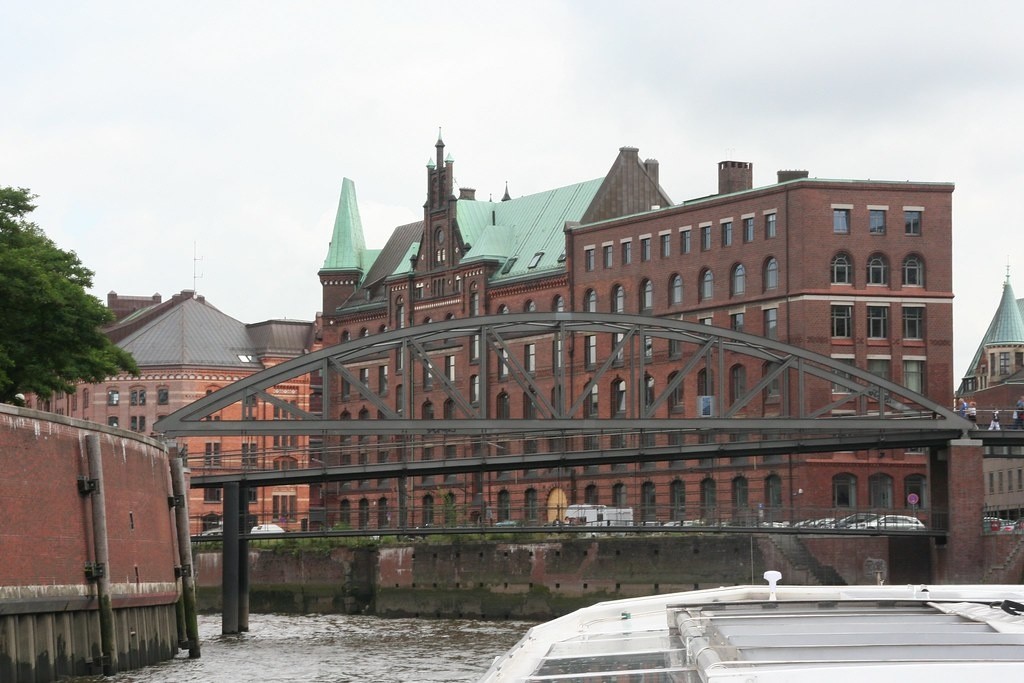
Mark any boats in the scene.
[482,570,1024,683]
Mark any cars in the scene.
[638,513,928,534]
[250,524,286,532]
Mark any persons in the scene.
[1012,395,1024,427]
[553,515,560,526]
[967,401,980,429]
[958,397,967,416]
[563,517,570,526]
[987,404,1000,430]
